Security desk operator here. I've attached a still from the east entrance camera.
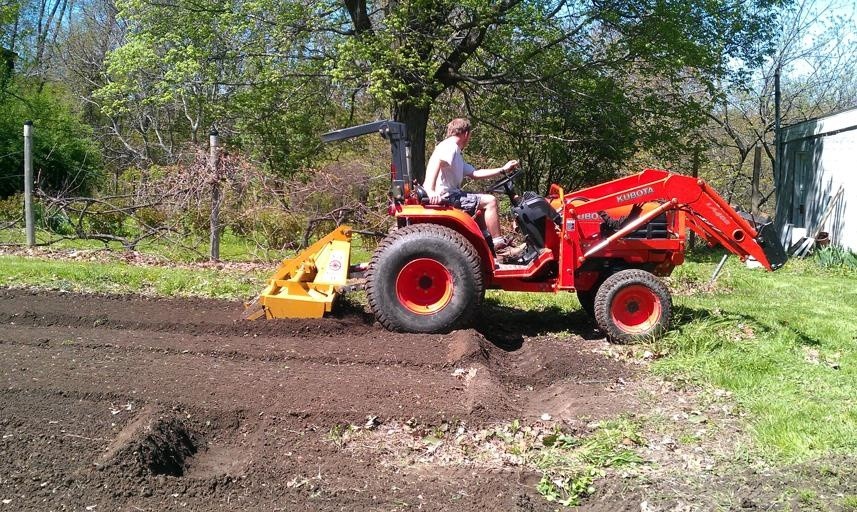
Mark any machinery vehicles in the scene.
[243,120,790,344]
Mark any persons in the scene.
[422,118,527,263]
